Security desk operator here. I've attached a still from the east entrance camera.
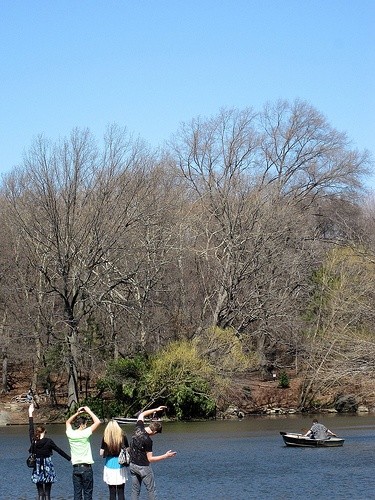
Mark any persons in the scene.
[99,421,130,500]
[303,419,337,439]
[129,406,177,500]
[28,404,71,500]
[65,406,101,500]
[27,387,35,400]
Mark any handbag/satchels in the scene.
[26,454,36,468]
[119,448,131,466]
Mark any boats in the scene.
[280,431,345,447]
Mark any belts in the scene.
[73,464,91,467]
